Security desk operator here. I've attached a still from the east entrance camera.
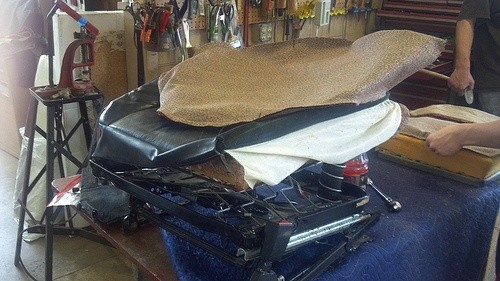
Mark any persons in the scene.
[446,0,500,116]
[426,116,500,159]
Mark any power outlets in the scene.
[253,24,274,42]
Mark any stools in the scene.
[15,83,115,280]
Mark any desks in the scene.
[51,148,500,281]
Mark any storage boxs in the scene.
[378,135,499,185]
[82,168,135,222]
[51,174,92,229]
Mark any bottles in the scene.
[347,153,369,211]
